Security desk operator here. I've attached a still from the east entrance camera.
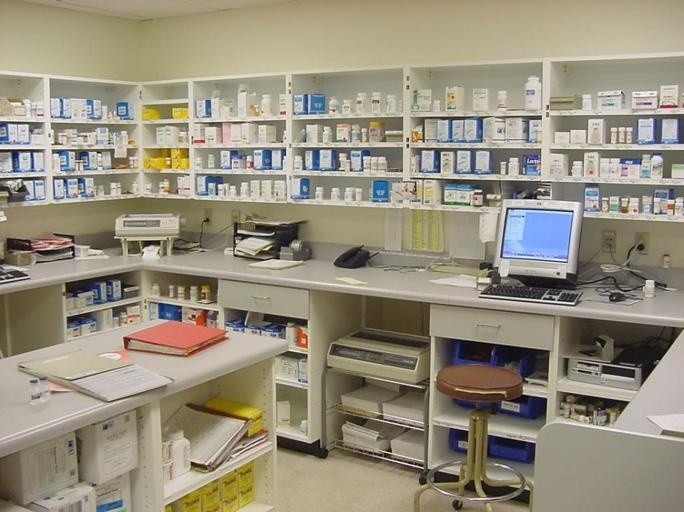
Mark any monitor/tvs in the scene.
[492,198,585,289]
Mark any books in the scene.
[18,347,175,401]
[249,258,303,270]
[164,402,268,473]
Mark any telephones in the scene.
[334,245,380,269]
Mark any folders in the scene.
[123,320,229,357]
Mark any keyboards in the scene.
[478,284,583,306]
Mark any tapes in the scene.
[292,238,303,251]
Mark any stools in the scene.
[412,365,534,512]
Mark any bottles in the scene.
[31,102,36,116]
[338,153,348,170]
[363,156,371,172]
[611,127,617,144]
[626,127,632,143]
[108,112,112,119]
[508,158,520,174]
[412,90,419,110]
[113,111,117,119]
[196,157,202,168]
[618,127,626,143]
[76,160,83,171]
[572,161,583,177]
[240,182,249,198]
[331,187,340,200]
[473,189,484,206]
[582,95,592,111]
[166,429,191,479]
[371,156,378,172]
[52,154,60,171]
[246,156,253,169]
[653,198,661,214]
[110,183,122,195]
[150,283,212,303]
[432,100,441,112]
[294,155,303,170]
[97,153,102,166]
[500,161,507,175]
[51,129,55,144]
[62,133,67,144]
[96,184,104,195]
[248,105,260,115]
[36,102,43,116]
[330,96,339,117]
[379,156,387,172]
[165,157,172,168]
[261,94,273,113]
[645,280,655,298]
[72,108,79,119]
[23,99,31,115]
[283,156,287,171]
[341,100,353,116]
[650,153,663,178]
[322,126,333,143]
[344,187,354,201]
[352,125,360,142]
[386,95,396,113]
[355,93,367,116]
[120,130,128,145]
[80,109,87,119]
[129,157,138,168]
[497,90,507,108]
[524,77,541,111]
[208,153,215,167]
[361,128,368,143]
[315,186,323,200]
[371,92,382,115]
[675,197,684,216]
[228,185,236,197]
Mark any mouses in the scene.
[609,291,626,302]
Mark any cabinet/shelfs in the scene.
[1,51,684,224]
[0,229,684,512]
[0,318,289,512]
